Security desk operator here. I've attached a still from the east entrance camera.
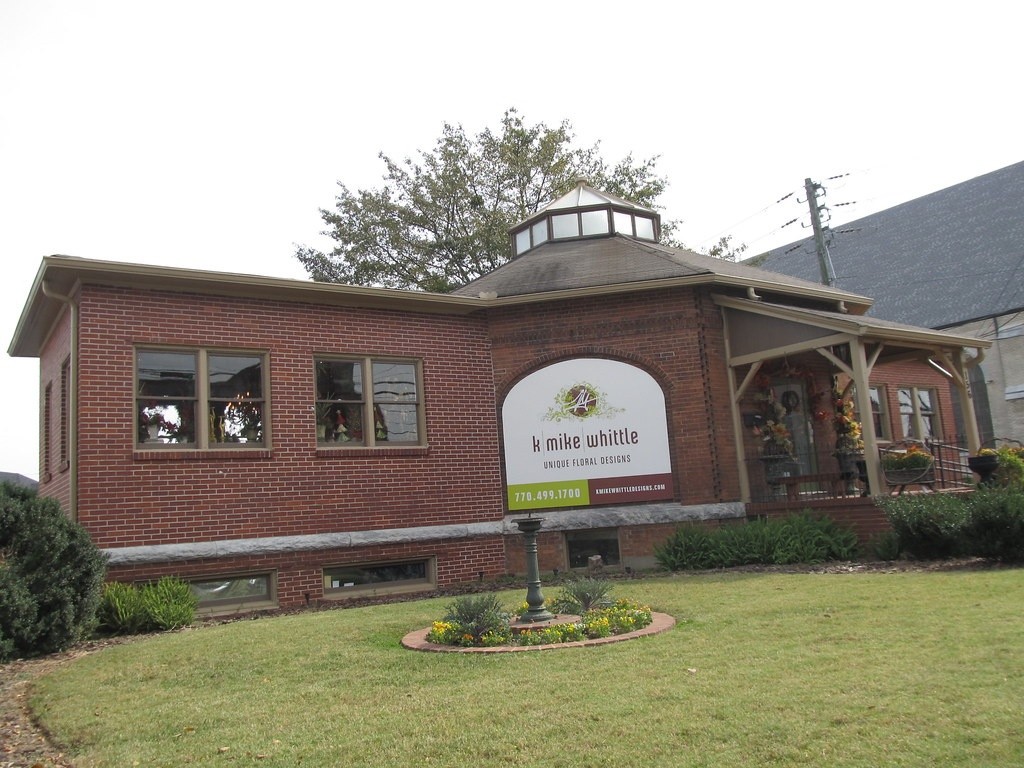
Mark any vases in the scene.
[761,454,794,502]
[967,456,999,470]
[884,468,936,484]
[835,450,866,493]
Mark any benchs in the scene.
[769,470,855,500]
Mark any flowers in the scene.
[746,374,795,463]
[977,447,1022,456]
[832,392,864,454]
[883,445,935,469]
